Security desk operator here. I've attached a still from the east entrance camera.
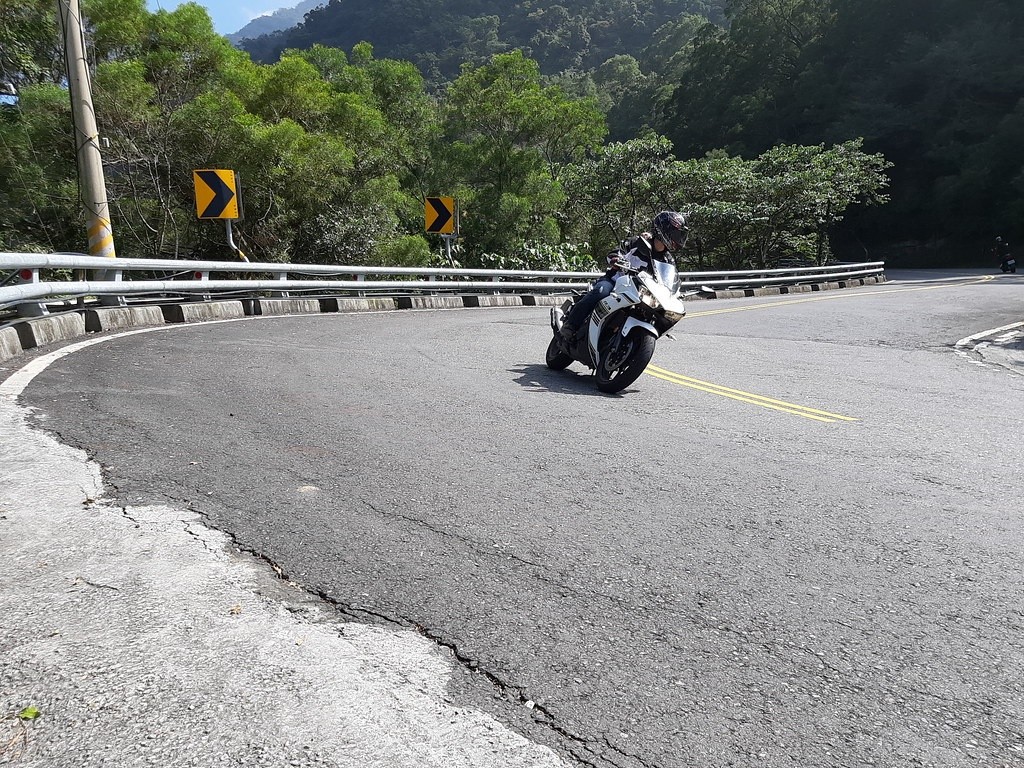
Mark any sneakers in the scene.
[561,319,578,339]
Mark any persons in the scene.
[559,211,687,338]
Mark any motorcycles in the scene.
[991,243,1016,272]
[546,247,687,395]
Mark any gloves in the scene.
[610,257,625,270]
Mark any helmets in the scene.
[651,211,690,253]
